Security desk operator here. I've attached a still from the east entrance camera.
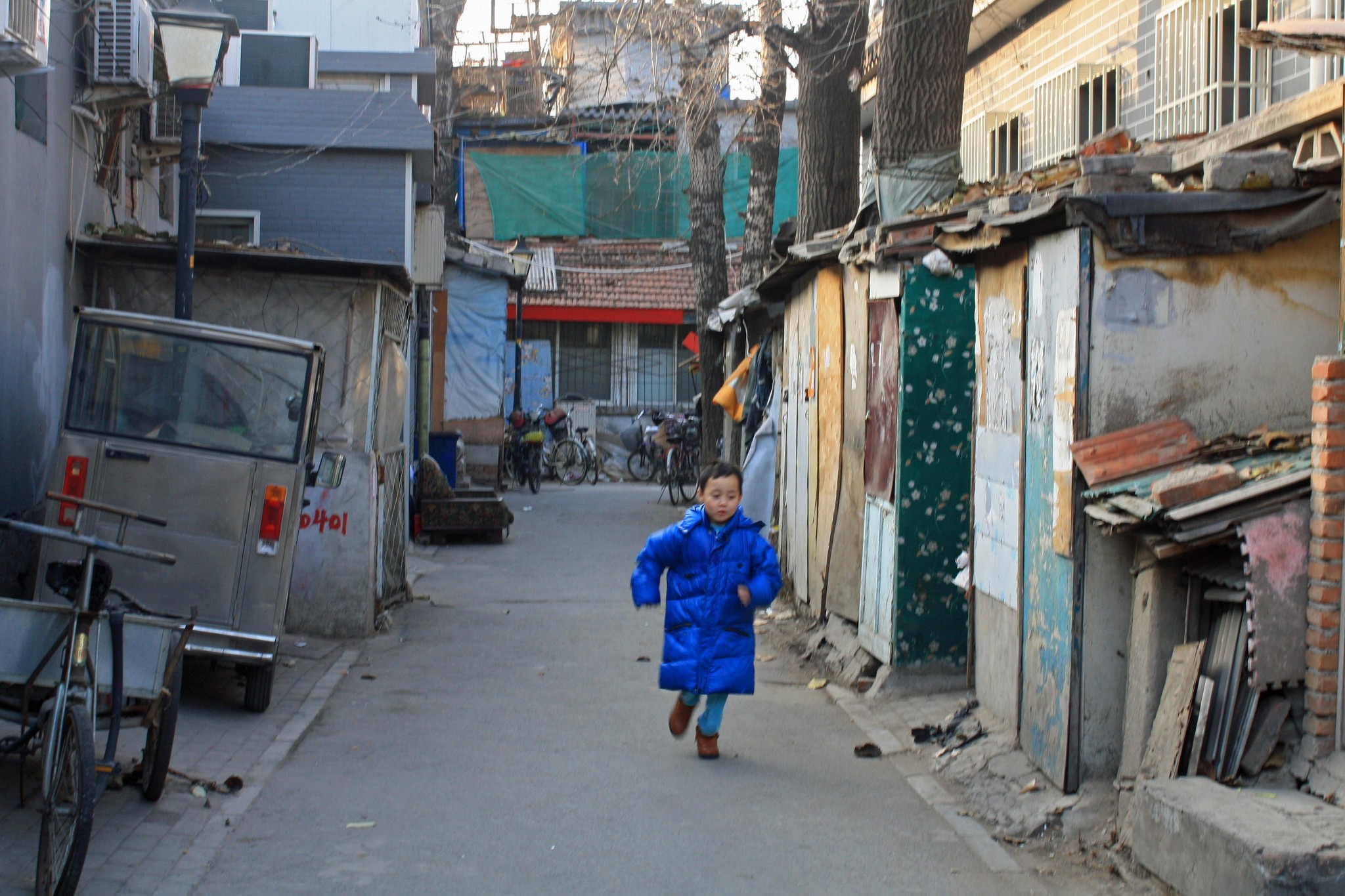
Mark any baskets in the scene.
[621,429,641,452]
[550,419,567,440]
[684,424,700,441]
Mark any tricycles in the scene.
[1,489,194,896]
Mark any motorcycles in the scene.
[31,300,329,715]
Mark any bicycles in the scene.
[620,406,700,506]
[505,403,599,496]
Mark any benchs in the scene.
[420,456,504,540]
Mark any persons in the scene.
[630,462,784,759]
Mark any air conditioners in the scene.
[148,79,202,145]
[94,0,155,88]
[0,0,50,65]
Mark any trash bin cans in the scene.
[414,431,461,489]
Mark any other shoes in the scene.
[669,699,695,736]
[696,726,719,758]
